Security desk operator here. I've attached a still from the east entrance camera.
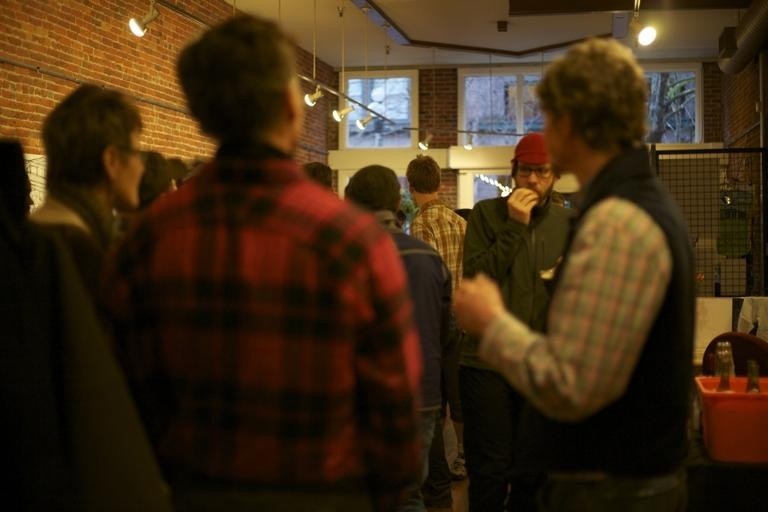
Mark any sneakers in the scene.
[454,458,467,480]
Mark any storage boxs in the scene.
[690,367,767,466]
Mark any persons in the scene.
[0,13,696,512]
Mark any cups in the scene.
[713,341,736,377]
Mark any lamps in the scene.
[126,1,164,38]
[289,0,401,136]
[409,36,489,153]
[626,1,658,47]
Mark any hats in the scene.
[511,132,549,165]
[139,152,187,204]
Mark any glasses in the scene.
[519,165,552,178]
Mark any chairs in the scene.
[697,326,768,380]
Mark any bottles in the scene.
[745,354,760,394]
